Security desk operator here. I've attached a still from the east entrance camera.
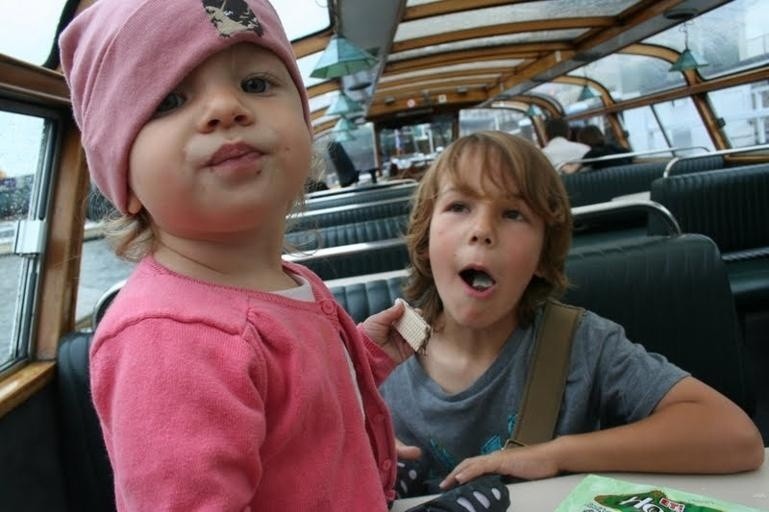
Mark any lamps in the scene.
[524,85,542,116]
[308,0,380,143]
[668,19,709,72]
[577,66,602,102]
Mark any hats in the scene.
[58,0,314,218]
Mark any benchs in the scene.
[55,145,769,512]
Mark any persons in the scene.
[374,128,767,500]
[55,0,431,511]
[541,115,634,176]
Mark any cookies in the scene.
[389,296,431,355]
[471,274,493,290]
